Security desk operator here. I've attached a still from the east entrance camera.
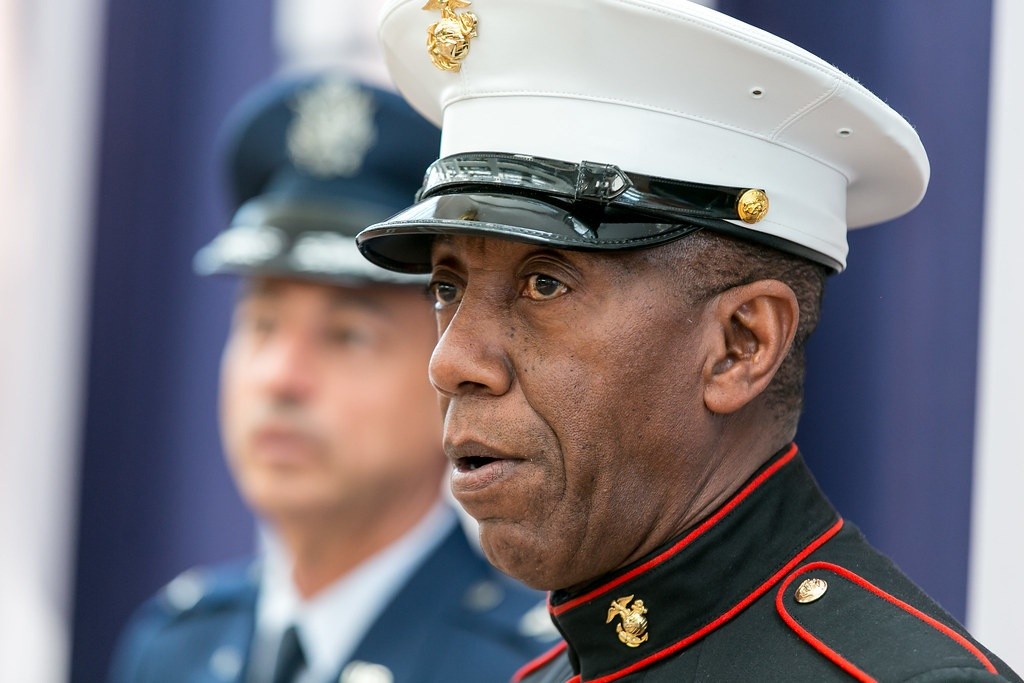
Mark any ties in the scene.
[273,625,309,683]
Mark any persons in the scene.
[108,62,575,683]
[355,0,1024,683]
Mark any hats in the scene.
[195,65,442,283]
[356,0,932,274]
